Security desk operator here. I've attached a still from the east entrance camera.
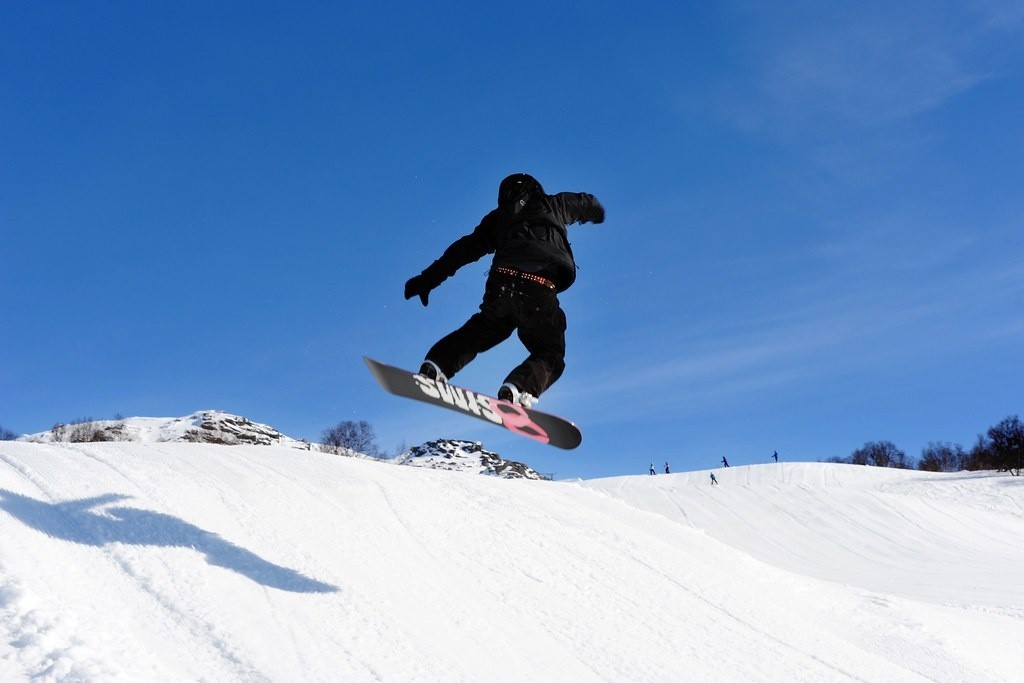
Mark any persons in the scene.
[721,456,729,467]
[404,174,605,410]
[710,473,718,484]
[664,461,670,473]
[772,450,777,462]
[650,462,655,475]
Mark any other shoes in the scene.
[417,360,448,383]
[498,383,538,410]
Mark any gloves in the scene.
[404,275,431,306]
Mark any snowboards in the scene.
[363,355,583,450]
